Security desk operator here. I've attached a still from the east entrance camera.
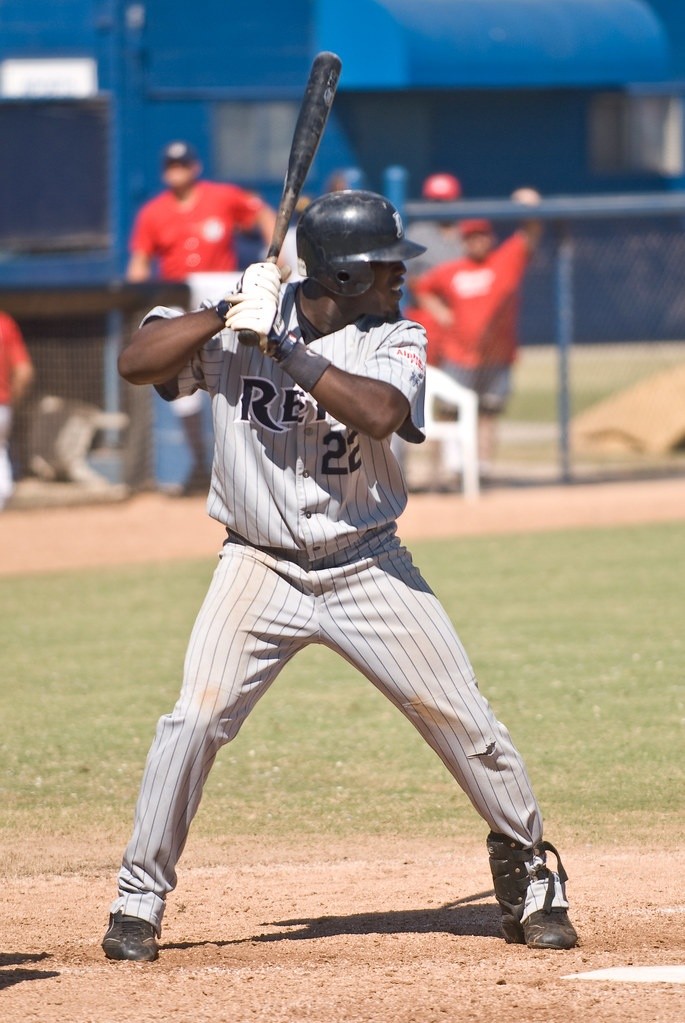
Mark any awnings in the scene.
[316,0,667,96]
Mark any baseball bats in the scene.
[236,51,345,349]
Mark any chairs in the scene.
[390,361,482,496]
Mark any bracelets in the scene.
[280,341,332,391]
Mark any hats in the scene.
[462,219,490,236]
[425,173,461,199]
[161,140,195,167]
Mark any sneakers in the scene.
[502,906,578,949]
[102,910,159,962]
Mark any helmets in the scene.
[295,189,427,297]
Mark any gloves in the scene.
[215,262,282,326]
[224,292,299,363]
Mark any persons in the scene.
[100,190,579,962]
[0,310,33,510]
[127,143,287,497]
[402,171,544,493]
